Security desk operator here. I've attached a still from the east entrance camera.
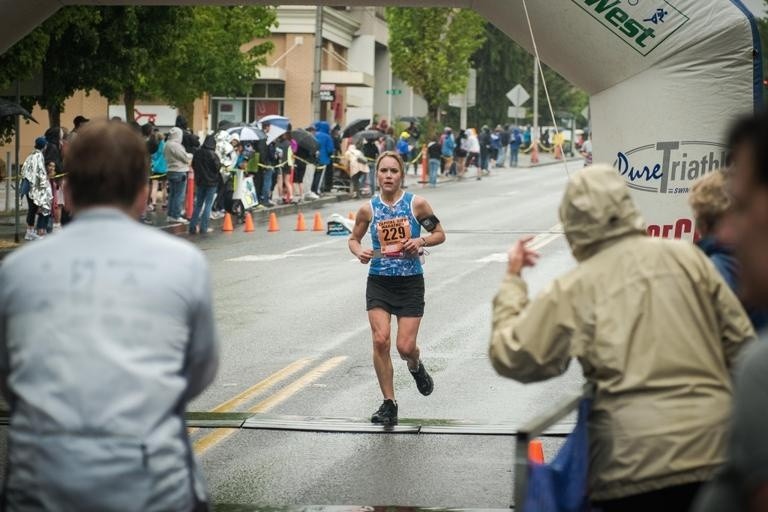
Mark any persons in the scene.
[487,161,761,512]
[0,114,225,512]
[691,113,768,512]
[19,115,597,237]
[689,168,766,333]
[347,148,447,429]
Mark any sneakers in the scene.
[25,229,40,240]
[263,199,304,206]
[371,399,397,422]
[409,361,433,395]
[167,211,226,233]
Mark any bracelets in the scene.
[422,237,428,248]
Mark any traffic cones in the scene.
[296,211,307,231]
[243,212,254,232]
[312,210,325,231]
[222,213,234,231]
[267,212,279,231]
[347,210,354,220]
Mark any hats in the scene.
[74,116,89,123]
[36,137,48,144]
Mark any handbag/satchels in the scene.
[358,158,368,164]
[21,179,29,199]
